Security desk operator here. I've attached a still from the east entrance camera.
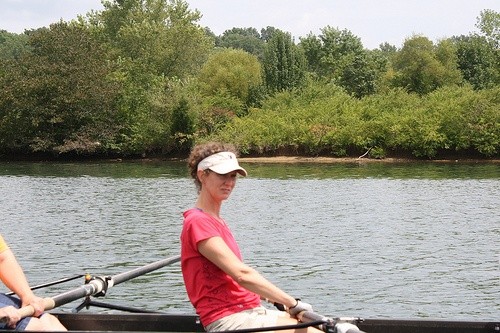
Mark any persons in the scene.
[0,235,68,331]
[179,140,326,333]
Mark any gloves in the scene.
[273,298,313,320]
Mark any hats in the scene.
[196,151,250,177]
